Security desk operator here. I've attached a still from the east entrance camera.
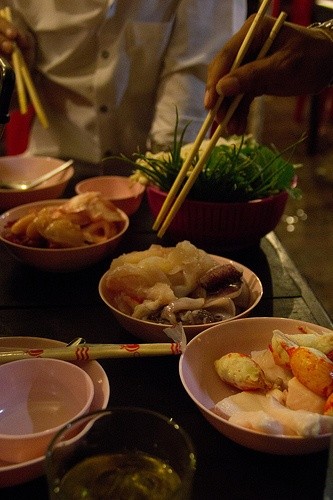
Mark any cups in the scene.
[41,406,197,500]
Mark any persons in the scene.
[0,0,248,180]
[203,13,333,136]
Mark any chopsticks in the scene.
[0,343,182,364]
[0,7,49,128]
[152,0,286,239]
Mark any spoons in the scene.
[0,159,74,192]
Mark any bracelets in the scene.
[307,19,333,42]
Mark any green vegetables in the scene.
[101,106,305,202]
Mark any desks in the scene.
[0,162,333,500]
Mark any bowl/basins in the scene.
[75,175,145,215]
[0,357,94,462]
[0,335,110,489]
[99,253,263,343]
[178,317,333,454]
[0,200,129,274]
[0,157,77,213]
[146,183,297,252]
